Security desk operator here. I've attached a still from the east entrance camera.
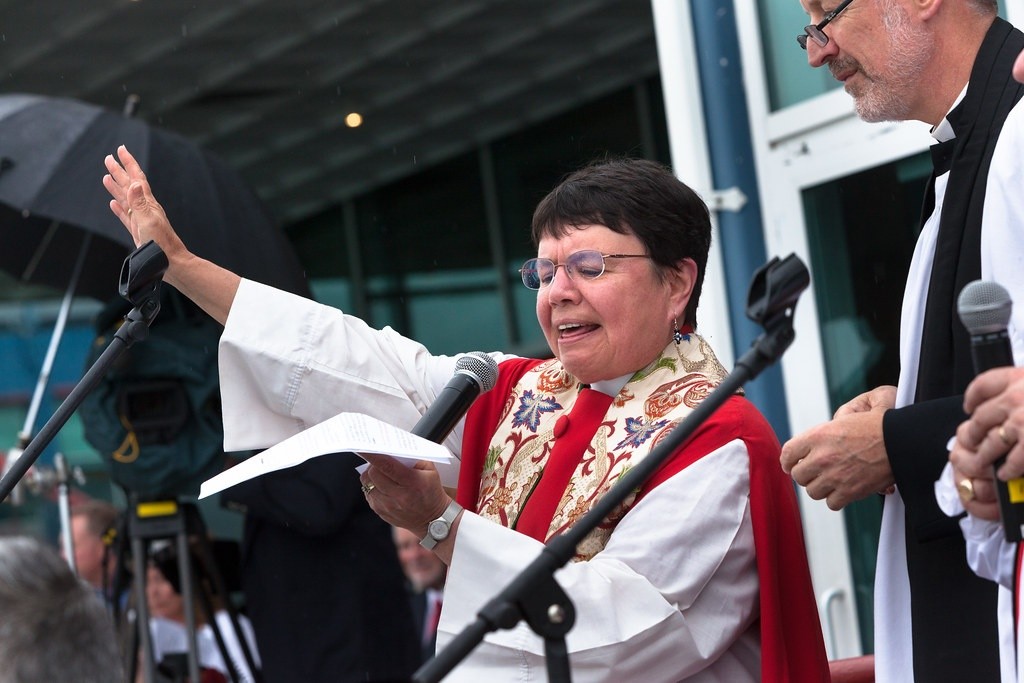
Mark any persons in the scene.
[934,56,1022,683]
[104,146,831,683]
[781,0,1024,683]
[0,300,449,683]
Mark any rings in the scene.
[127,209,133,218]
[361,481,375,494]
[960,477,976,502]
[999,427,1014,448]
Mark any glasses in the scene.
[796,0,852,50]
[519,250,655,290]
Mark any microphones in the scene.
[958,280,1024,544]
[410,351,499,446]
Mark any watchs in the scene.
[419,500,464,552]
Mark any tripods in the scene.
[113,497,262,683]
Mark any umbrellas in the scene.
[2,95,310,462]
